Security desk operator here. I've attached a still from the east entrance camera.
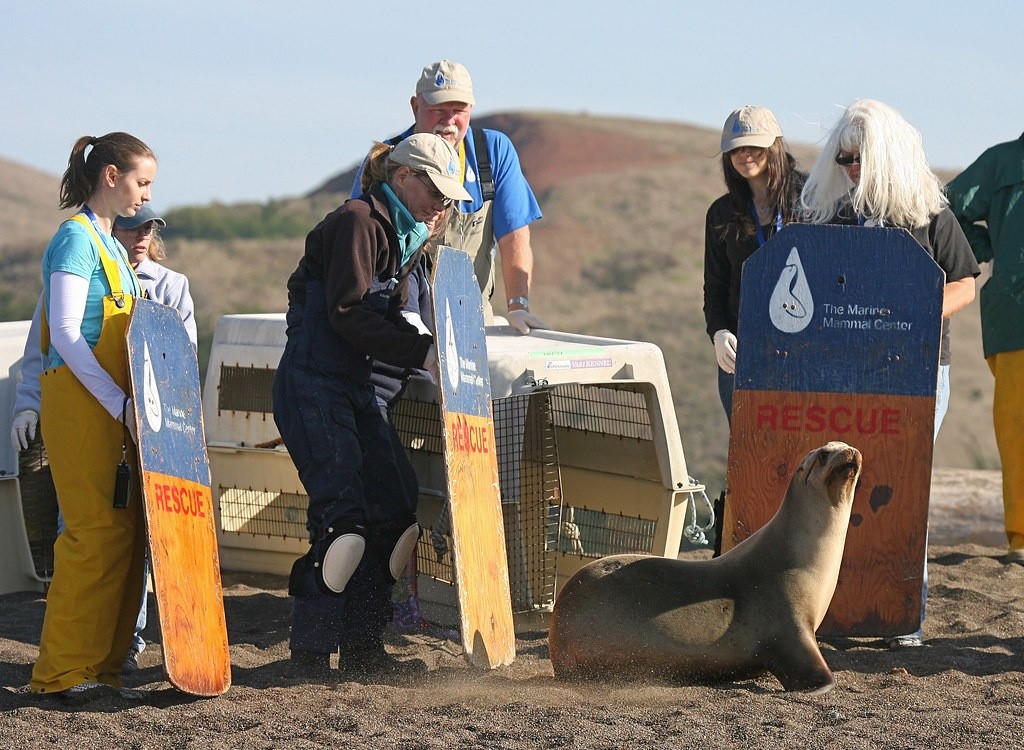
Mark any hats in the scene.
[389,133,474,203]
[115,205,167,229]
[721,104,784,154]
[415,59,476,106]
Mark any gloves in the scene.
[712,329,737,374]
[11,410,38,451]
[505,310,552,334]
[423,344,438,383]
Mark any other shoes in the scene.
[338,639,426,675]
[122,649,139,671]
[291,649,341,679]
[62,683,147,702]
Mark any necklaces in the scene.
[754,191,770,213]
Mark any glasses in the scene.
[835,151,860,165]
[415,175,452,205]
[116,229,151,240]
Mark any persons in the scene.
[703,105,811,428]
[12,201,198,671]
[272,132,473,683]
[29,133,163,704]
[800,98,981,647]
[940,132,1024,564]
[350,60,553,335]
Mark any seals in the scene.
[548,440,864,696]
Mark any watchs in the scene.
[508,297,529,309]
